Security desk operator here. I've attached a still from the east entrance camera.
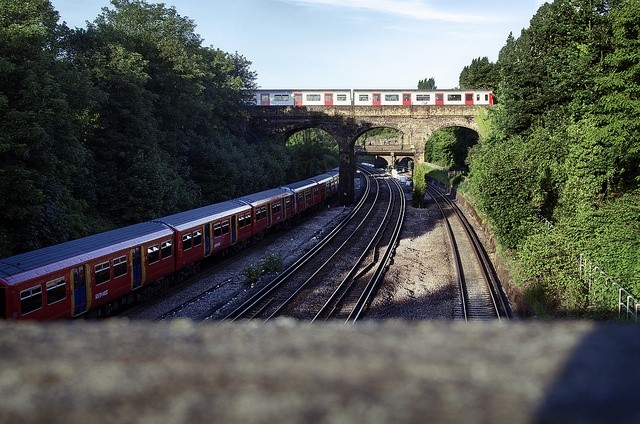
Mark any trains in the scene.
[0,166,339,321]
[241,88,494,106]
